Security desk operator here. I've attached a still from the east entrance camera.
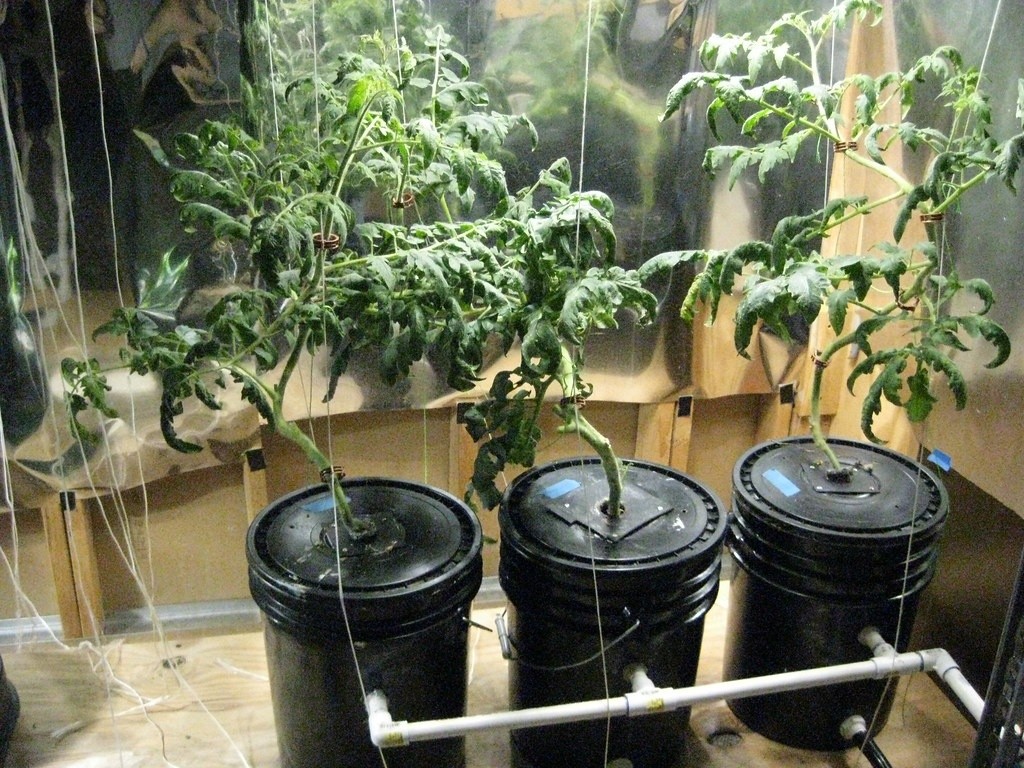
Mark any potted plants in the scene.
[51,0,1024,768]
